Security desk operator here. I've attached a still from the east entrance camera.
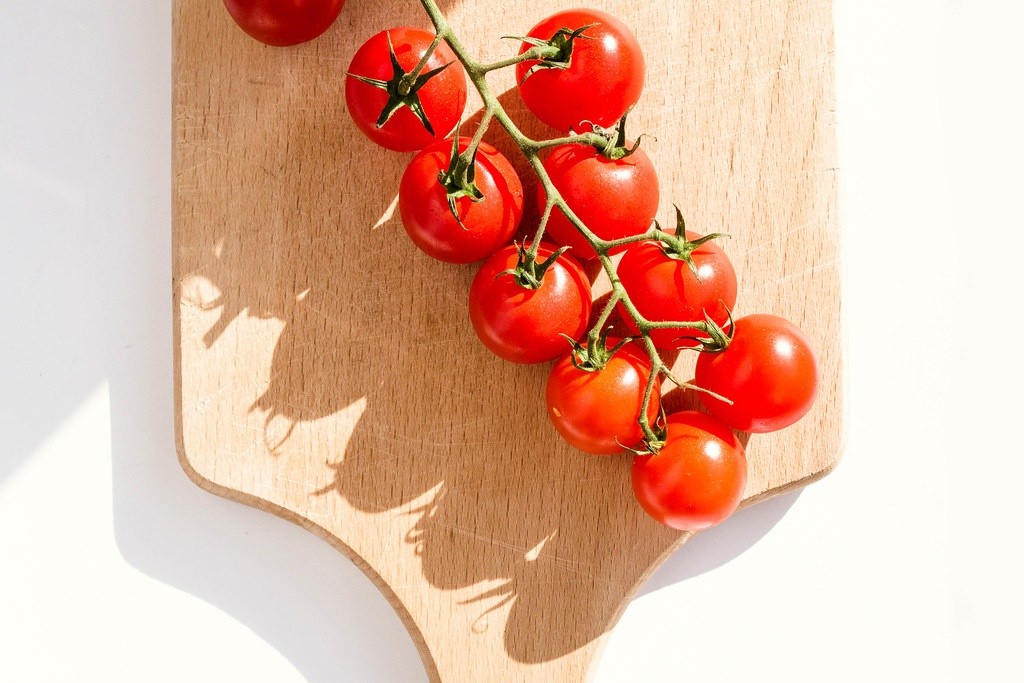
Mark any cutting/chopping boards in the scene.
[172,0,840,683]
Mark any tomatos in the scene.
[223,0,346,46]
[345,8,821,532]
